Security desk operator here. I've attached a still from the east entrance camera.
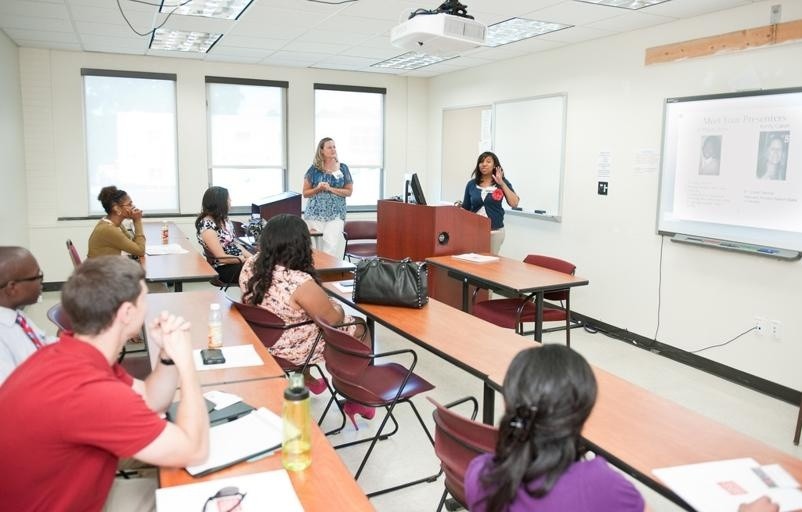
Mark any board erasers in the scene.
[535,210,546,214]
[512,208,522,211]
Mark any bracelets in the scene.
[157,356,175,366]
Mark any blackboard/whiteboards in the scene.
[653,84,797,264]
[490,92,569,223]
[440,106,490,202]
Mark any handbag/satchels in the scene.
[352,256,428,308]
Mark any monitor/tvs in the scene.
[410,174,426,205]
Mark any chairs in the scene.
[67,240,81,268]
[47,303,151,380]
[230,220,246,242]
[470,255,578,335]
[224,296,367,436]
[426,395,502,512]
[195,236,242,295]
[343,221,377,264]
[316,318,443,499]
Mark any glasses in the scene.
[0,270,43,289]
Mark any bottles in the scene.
[281,374,312,472]
[208,304,223,349]
[161,220,169,240]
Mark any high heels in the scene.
[306,378,327,394]
[344,400,375,430]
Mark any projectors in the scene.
[390,13,487,57]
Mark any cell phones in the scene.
[200,348,226,365]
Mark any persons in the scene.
[699,136,719,176]
[0,254,210,512]
[464,344,782,512]
[303,137,353,260]
[195,186,255,284]
[238,213,376,431]
[757,136,787,180]
[88,186,146,343]
[0,246,60,387]
[461,151,519,300]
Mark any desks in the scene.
[426,250,586,340]
[488,354,802,512]
[137,290,287,387]
[156,377,378,512]
[311,249,356,286]
[141,221,219,292]
[322,279,540,390]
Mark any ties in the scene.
[16,315,43,349]
